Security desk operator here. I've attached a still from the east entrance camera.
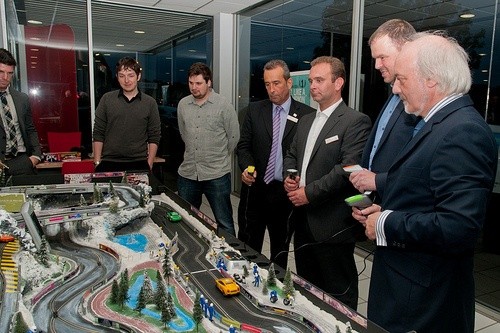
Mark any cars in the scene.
[215,278,240,297]
[165,211,182,222]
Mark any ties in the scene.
[412,119,425,138]
[263,105,283,184]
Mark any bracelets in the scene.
[93,160,101,166]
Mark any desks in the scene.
[36,157,165,183]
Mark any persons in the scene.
[348,19,498,333]
[92,57,161,172]
[176,63,240,237]
[235,61,318,270]
[283,57,372,312]
[0,49,42,187]
